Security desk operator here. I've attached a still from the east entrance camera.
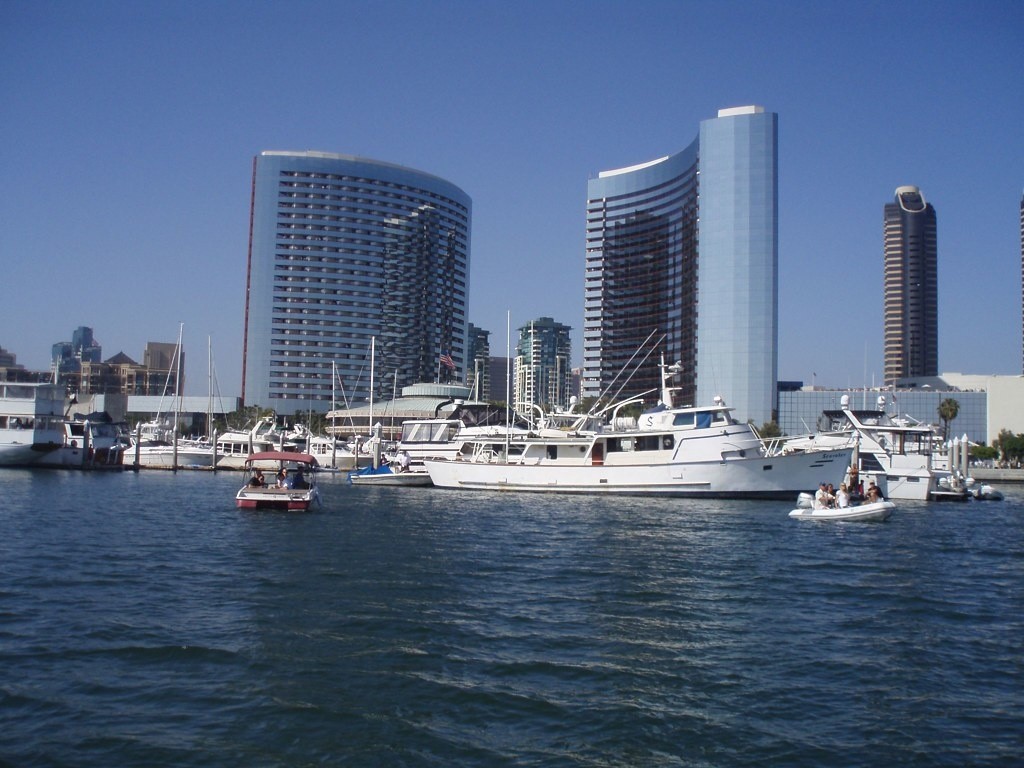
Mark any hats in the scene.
[819,481,827,486]
[869,480,874,484]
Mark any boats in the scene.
[235,451,321,514]
[788,492,897,523]
[0,310,1006,500]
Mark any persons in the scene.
[957,466,964,485]
[395,451,411,472]
[813,481,849,511]
[292,466,309,489]
[380,453,391,467]
[276,468,287,487]
[247,468,265,488]
[994,457,1017,469]
[850,463,884,506]
[352,464,359,471]
